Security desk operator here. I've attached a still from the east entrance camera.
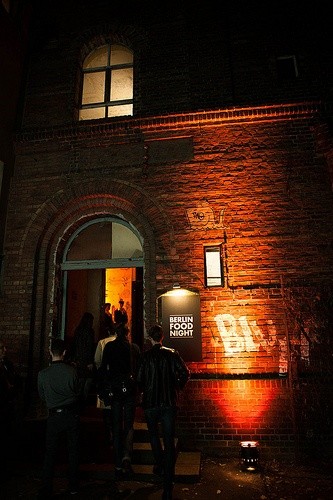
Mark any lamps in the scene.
[173,283,181,289]
[204,242,224,288]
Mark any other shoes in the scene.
[68,478,80,496]
[113,468,123,481]
[162,488,172,500]
[122,456,134,475]
[32,468,52,500]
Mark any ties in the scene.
[0,362,16,390]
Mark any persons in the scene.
[74,301,128,463]
[0,343,12,478]
[100,324,144,477]
[138,324,189,474]
[37,339,85,496]
